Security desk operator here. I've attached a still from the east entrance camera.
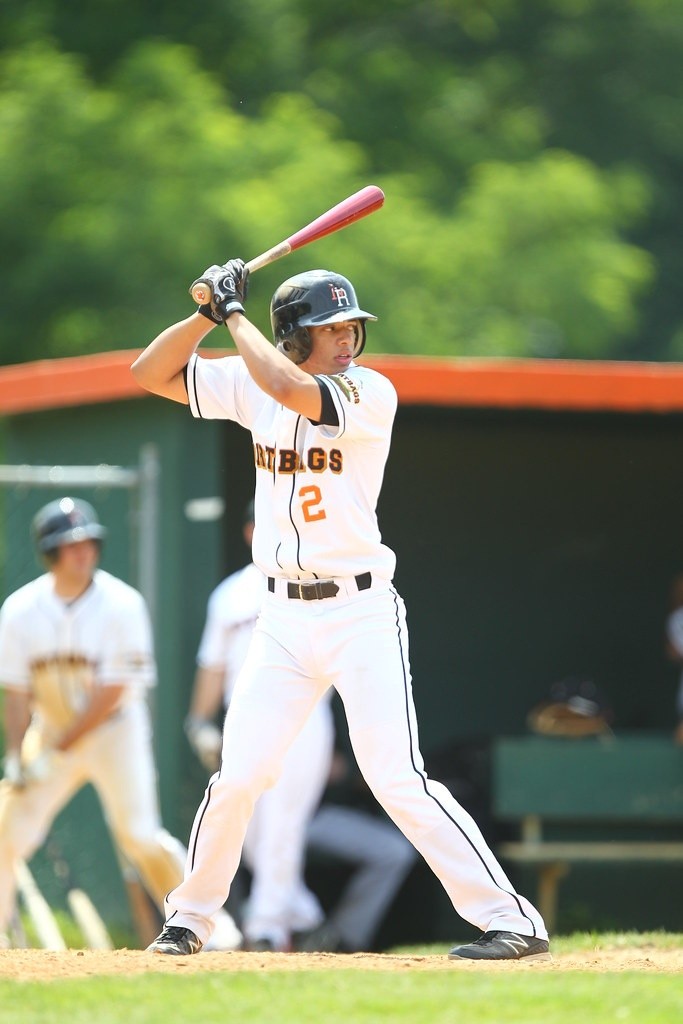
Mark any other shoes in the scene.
[201,914,242,950]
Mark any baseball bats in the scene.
[192,185,385,305]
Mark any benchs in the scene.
[491,737,683,939]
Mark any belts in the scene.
[269,572,372,599]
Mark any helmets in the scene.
[269,270,378,365]
[31,496,105,553]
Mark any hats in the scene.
[244,499,254,522]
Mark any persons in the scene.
[184,499,334,953]
[301,751,415,957]
[129,257,549,966]
[1,497,243,948]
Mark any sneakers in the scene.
[146,927,202,955]
[447,930,552,961]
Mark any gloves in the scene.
[188,257,248,326]
[31,750,69,780]
[3,755,29,785]
